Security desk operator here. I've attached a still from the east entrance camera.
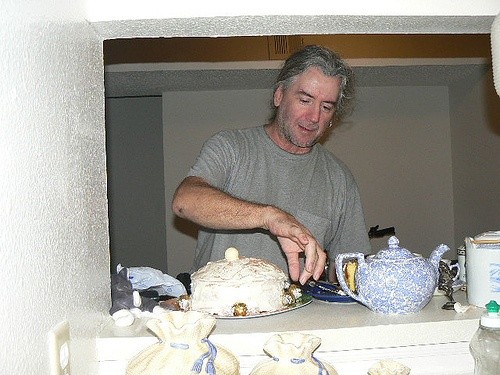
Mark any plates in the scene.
[305,278,361,302]
[183,289,313,319]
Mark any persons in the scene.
[171,44,373,292]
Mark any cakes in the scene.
[178,247,303,316]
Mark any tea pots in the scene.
[334,235,451,315]
[465,230,500,307]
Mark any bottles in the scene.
[470,300,500,375]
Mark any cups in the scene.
[440,258,460,284]
